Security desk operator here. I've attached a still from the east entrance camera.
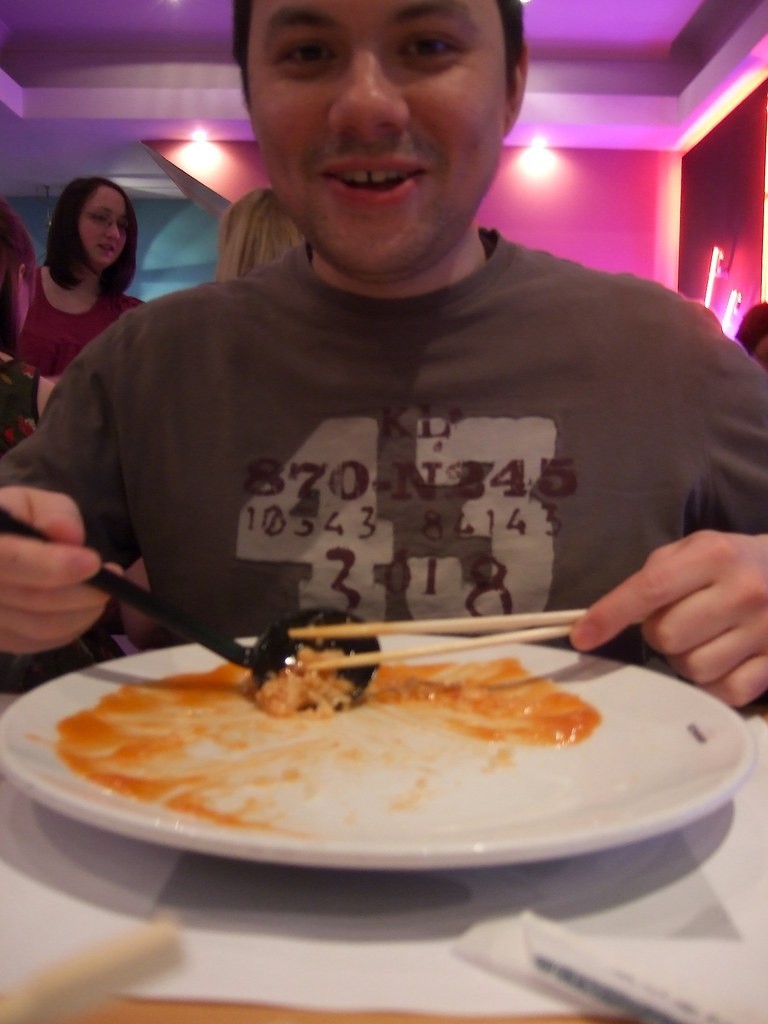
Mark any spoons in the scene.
[1,506,380,710]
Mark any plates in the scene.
[0,633,757,871]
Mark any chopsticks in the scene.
[288,608,588,673]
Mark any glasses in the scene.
[77,207,130,235]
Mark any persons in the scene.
[216,187,302,282]
[0,0,768,709]
[16,177,146,378]
[735,301,768,369]
[0,191,55,459]
